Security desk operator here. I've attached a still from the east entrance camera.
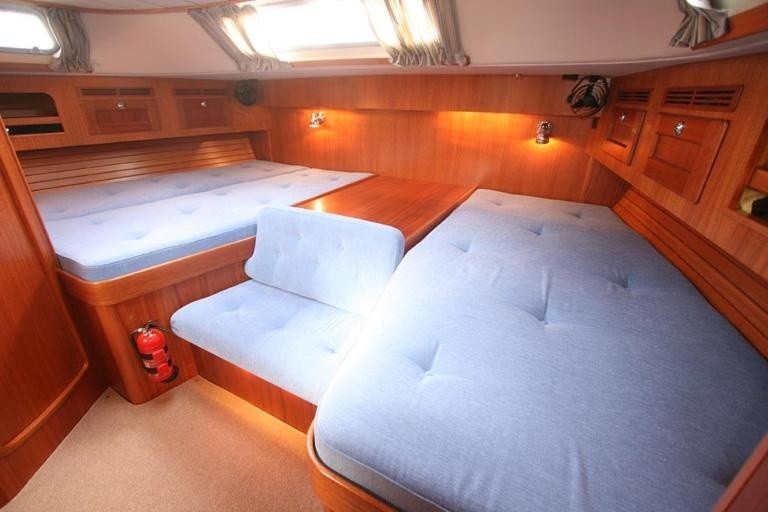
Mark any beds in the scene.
[307,188,768,512]
[31,157,377,405]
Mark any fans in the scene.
[235,79,257,105]
[567,75,609,116]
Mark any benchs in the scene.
[166,203,406,433]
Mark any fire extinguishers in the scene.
[129,320,175,383]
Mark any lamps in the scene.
[308,112,325,128]
[535,120,553,144]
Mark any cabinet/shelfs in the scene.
[0,72,230,152]
[599,51,768,286]
